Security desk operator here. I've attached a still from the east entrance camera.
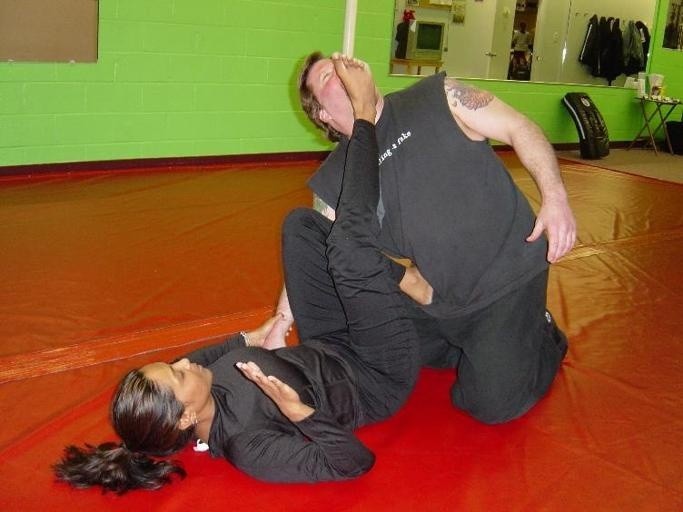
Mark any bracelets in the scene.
[239,331,251,347]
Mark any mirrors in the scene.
[388,0,656,89]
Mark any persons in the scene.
[513,22,531,50]
[51,52,406,496]
[265,51,577,424]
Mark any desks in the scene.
[390,58,444,75]
[626,97,683,157]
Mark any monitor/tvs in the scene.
[406,19,445,61]
